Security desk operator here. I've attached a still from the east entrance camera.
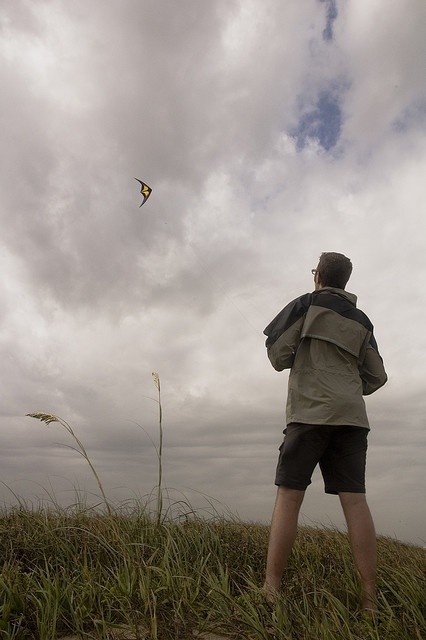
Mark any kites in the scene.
[133,177,152,208]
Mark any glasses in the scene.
[309,268,319,279]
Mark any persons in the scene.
[262,252,388,623]
